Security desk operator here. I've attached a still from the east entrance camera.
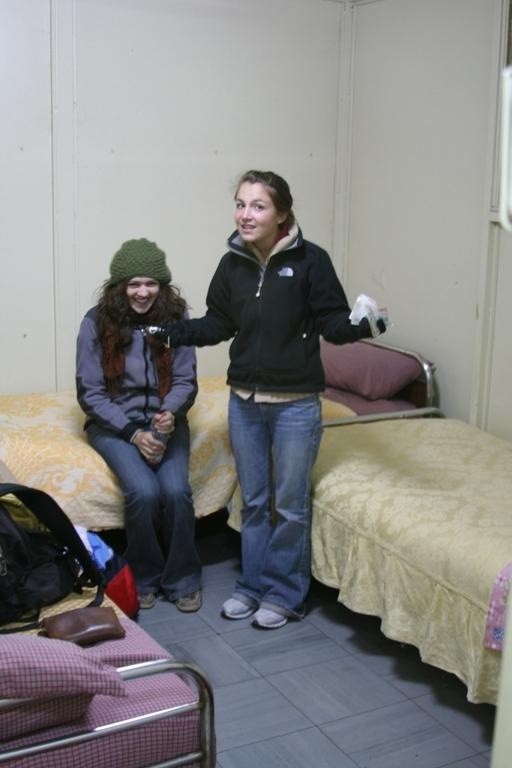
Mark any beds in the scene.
[1,578,225,768]
[231,405,510,765]
[0,334,441,531]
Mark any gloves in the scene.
[343,316,387,344]
[161,322,187,348]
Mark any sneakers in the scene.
[174,588,202,612]
[135,591,157,608]
[253,608,288,630]
[221,597,254,620]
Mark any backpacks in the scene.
[0,482,108,633]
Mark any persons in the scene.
[147,168,389,629]
[75,237,203,613]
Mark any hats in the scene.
[111,240,171,284]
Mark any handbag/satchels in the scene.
[82,530,137,620]
[41,606,125,645]
